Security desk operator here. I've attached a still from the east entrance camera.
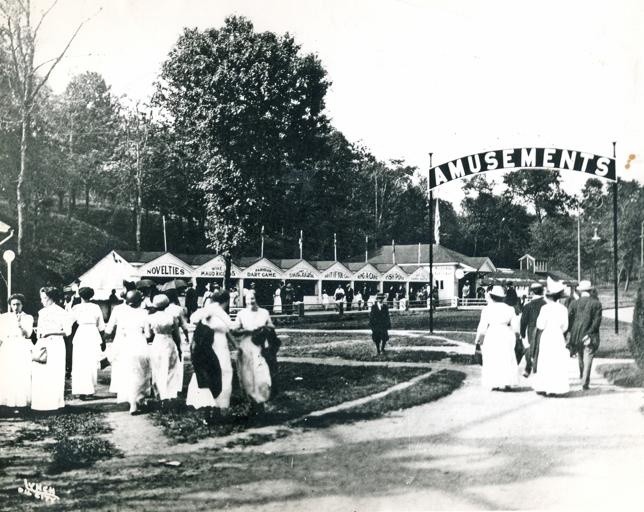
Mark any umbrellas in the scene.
[136,279,155,291]
[161,278,189,294]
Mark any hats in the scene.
[126,290,141,305]
[531,283,542,289]
[576,280,592,291]
[488,285,506,298]
[153,294,169,308]
[376,293,385,299]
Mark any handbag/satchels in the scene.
[32,348,46,364]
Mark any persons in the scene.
[186,281,200,314]
[213,283,240,307]
[368,293,391,355]
[464,279,471,297]
[244,280,307,314]
[321,289,330,310]
[569,279,603,390]
[186,291,235,408]
[346,283,354,310]
[536,276,569,397]
[333,284,345,305]
[504,285,525,366]
[202,281,212,307]
[462,282,470,298]
[361,283,370,310]
[69,287,105,400]
[388,284,439,308]
[475,285,521,391]
[0,294,33,414]
[106,287,121,316]
[145,295,182,406]
[164,288,189,361]
[230,290,275,411]
[104,288,149,415]
[521,283,548,378]
[31,286,72,413]
[356,290,363,311]
[60,281,79,317]
[476,284,486,299]
